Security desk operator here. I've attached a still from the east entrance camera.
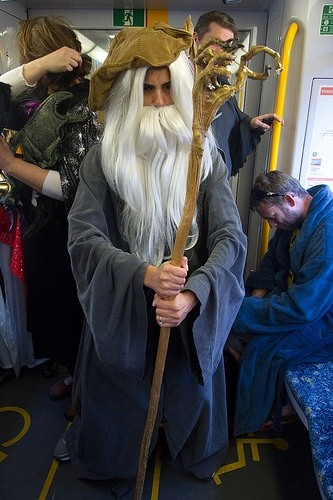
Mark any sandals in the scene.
[48,376,73,401]
[63,404,76,422]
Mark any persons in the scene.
[232,171,333,436]
[0,11,285,482]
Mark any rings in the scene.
[158,316,164,325]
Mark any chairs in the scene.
[285,362,333,500]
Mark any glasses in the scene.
[248,188,294,202]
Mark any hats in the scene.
[88,22,193,112]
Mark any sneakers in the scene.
[53,433,71,462]
